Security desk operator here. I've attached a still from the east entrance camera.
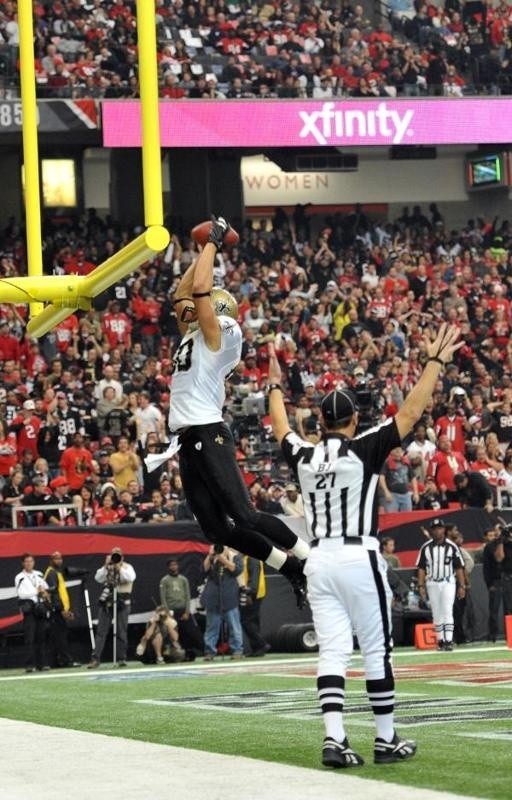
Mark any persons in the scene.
[317,362,469,403]
[317,322,468,367]
[244,335,313,367]
[415,518,466,652]
[226,368,260,408]
[14,554,49,671]
[236,296,313,334]
[260,322,467,756]
[283,368,315,446]
[223,254,315,296]
[161,558,191,662]
[0,0,512,99]
[41,548,74,667]
[379,437,467,515]
[452,529,474,644]
[85,548,137,669]
[443,521,458,552]
[237,222,313,257]
[464,323,512,514]
[384,405,467,458]
[167,214,315,612]
[169,229,206,309]
[1,206,179,529]
[239,553,267,657]
[264,462,305,518]
[236,433,261,464]
[472,527,497,564]
[482,525,512,643]
[270,203,315,243]
[199,542,245,661]
[317,200,509,323]
[136,605,179,667]
[380,535,400,566]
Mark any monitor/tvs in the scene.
[466,152,509,193]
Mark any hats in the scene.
[49,476,69,489]
[320,388,357,423]
[428,518,445,528]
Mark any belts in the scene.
[310,537,362,548]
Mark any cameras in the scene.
[109,553,122,565]
[214,544,224,554]
[64,566,89,577]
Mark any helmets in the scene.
[209,287,240,321]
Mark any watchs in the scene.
[263,383,283,393]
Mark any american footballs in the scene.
[191,221,240,248]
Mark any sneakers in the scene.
[319,736,365,769]
[293,558,310,611]
[436,639,445,651]
[374,733,419,764]
[447,639,454,651]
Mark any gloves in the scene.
[206,213,231,250]
